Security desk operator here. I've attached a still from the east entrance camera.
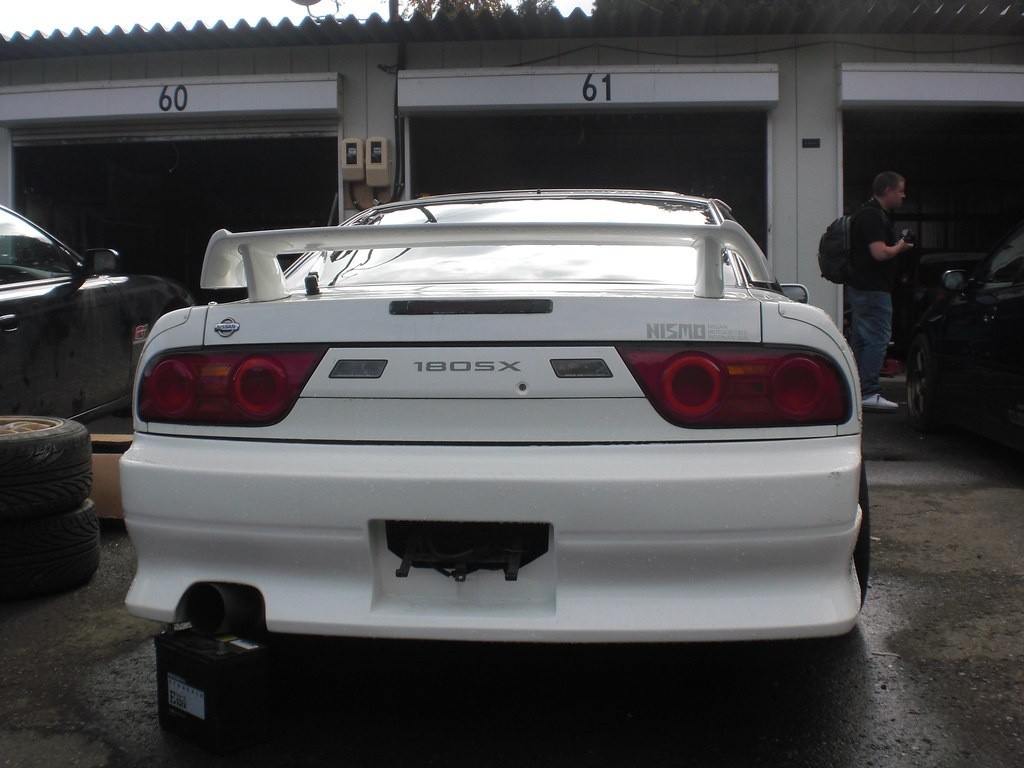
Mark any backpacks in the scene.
[818,206,891,284]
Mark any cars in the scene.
[843,219,1024,455]
[0,202,197,425]
[117,188,871,649]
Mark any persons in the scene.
[848,171,913,412]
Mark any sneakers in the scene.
[861,393,899,409]
